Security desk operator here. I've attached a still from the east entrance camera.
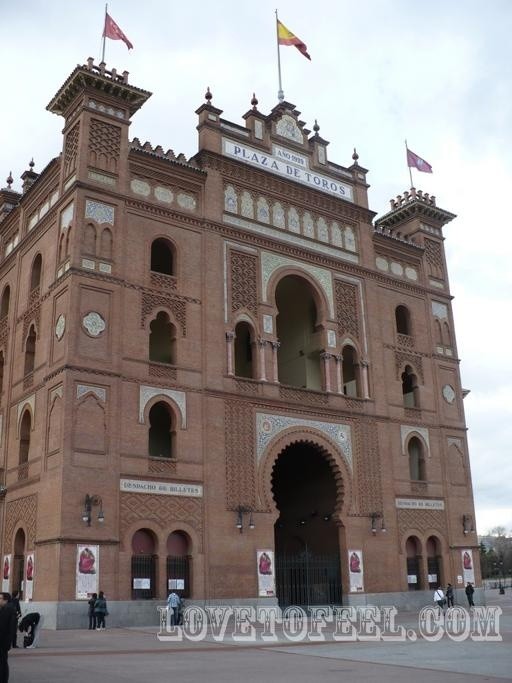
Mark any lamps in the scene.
[370,510,388,536]
[81,492,104,526]
[462,513,476,536]
[234,502,256,533]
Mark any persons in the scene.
[87,592,97,629]
[178,593,186,625]
[10,589,21,648]
[350,551,361,572]
[20,609,44,648]
[259,552,273,574]
[0,592,16,683]
[26,556,34,580]
[465,581,474,607]
[4,556,9,579]
[464,552,472,569]
[93,590,109,630]
[167,589,180,625]
[433,583,446,616]
[79,547,96,573]
[498,584,505,594]
[446,583,454,608]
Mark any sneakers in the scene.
[26,646,36,649]
[89,627,106,631]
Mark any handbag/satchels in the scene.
[441,599,446,603]
[24,636,33,647]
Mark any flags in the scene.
[276,18,311,61]
[103,12,134,49]
[406,147,432,173]
